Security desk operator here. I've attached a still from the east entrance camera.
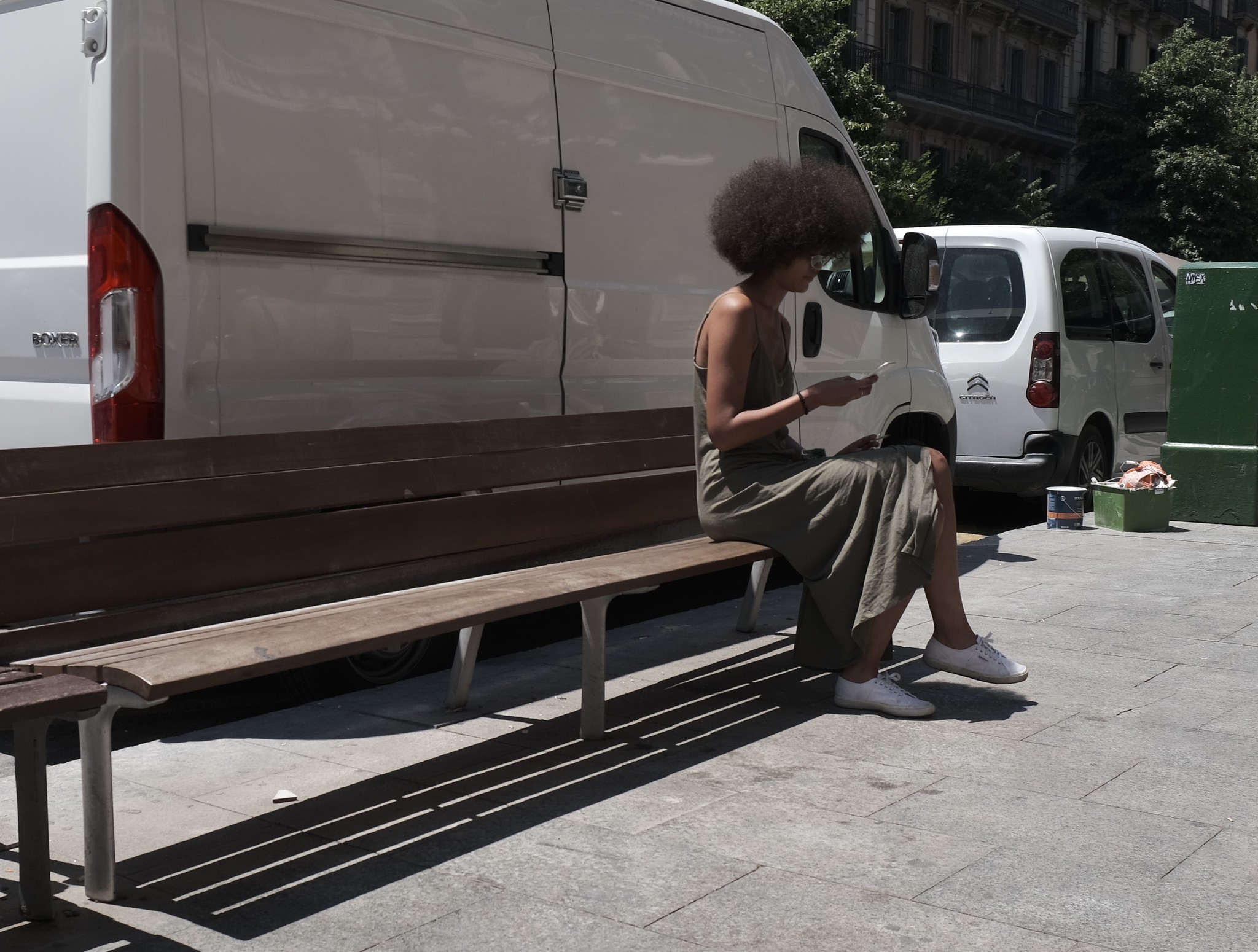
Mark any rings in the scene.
[860,388,864,398]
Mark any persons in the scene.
[692,153,1029,716]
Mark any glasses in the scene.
[804,254,833,267]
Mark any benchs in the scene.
[11,530,770,885]
[0,666,107,925]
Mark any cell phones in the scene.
[860,361,896,379]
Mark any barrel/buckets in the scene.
[1046,486,1087,530]
[1090,460,1177,532]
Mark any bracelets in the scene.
[797,391,810,415]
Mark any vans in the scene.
[821,222,1177,518]
[2,1,962,557]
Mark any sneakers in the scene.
[835,674,935,716]
[922,633,1029,683]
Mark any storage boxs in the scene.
[1090,481,1178,532]
